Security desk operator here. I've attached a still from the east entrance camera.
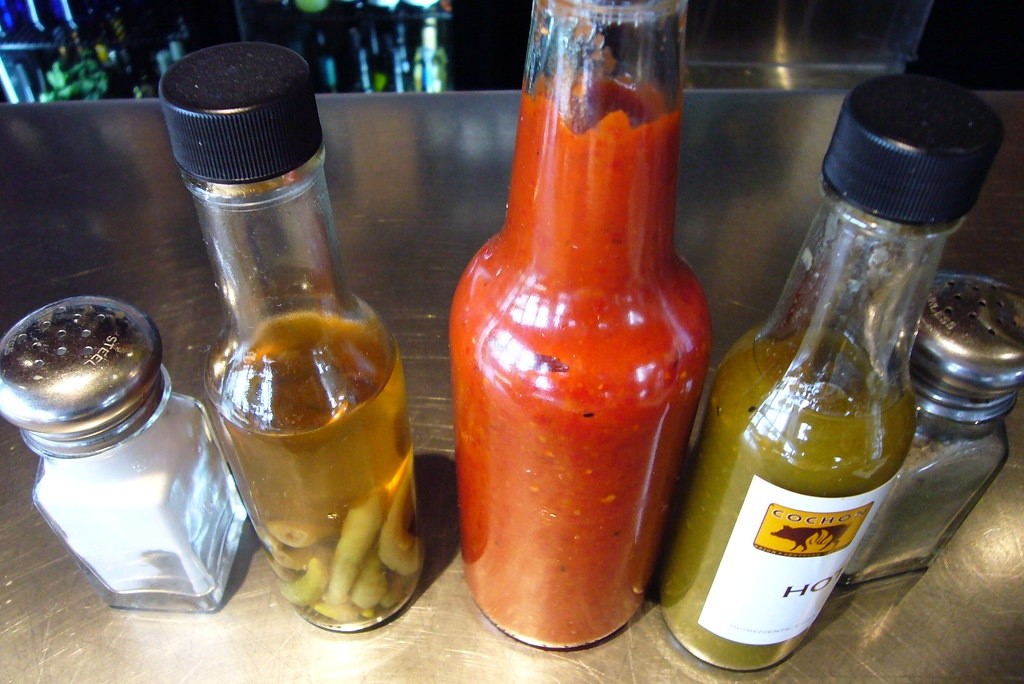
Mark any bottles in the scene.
[0,295,247,614]
[836,273,1024,584]
[448,0,712,650]
[657,72,1004,673]
[159,41,425,634]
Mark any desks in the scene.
[0,92,1024,684]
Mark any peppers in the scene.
[263,448,425,622]
